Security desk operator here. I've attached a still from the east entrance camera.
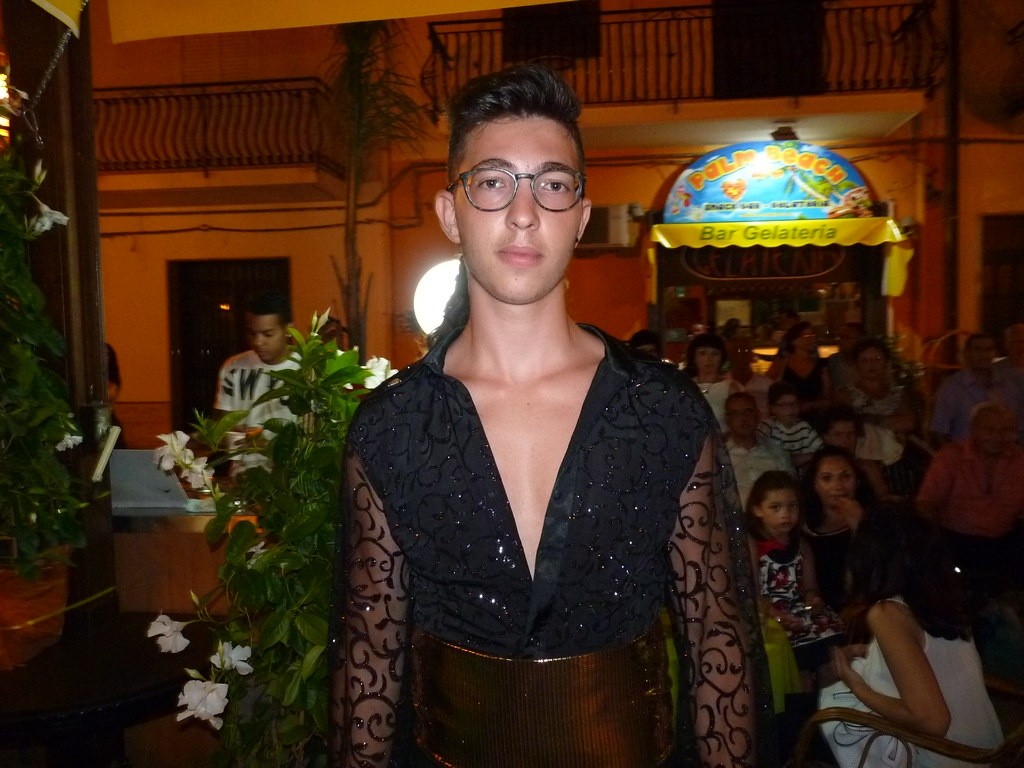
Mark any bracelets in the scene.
[775,610,786,622]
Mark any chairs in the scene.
[785,671,1024,768]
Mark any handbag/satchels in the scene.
[818,656,915,768]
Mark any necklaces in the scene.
[698,376,718,393]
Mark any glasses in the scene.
[446,166,586,212]
[777,403,798,408]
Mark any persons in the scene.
[105,341,123,448]
[327,62,783,768]
[628,317,1024,768]
[214,295,317,515]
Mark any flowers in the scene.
[144,310,408,768]
[0,152,96,583]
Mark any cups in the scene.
[224,431,247,460]
